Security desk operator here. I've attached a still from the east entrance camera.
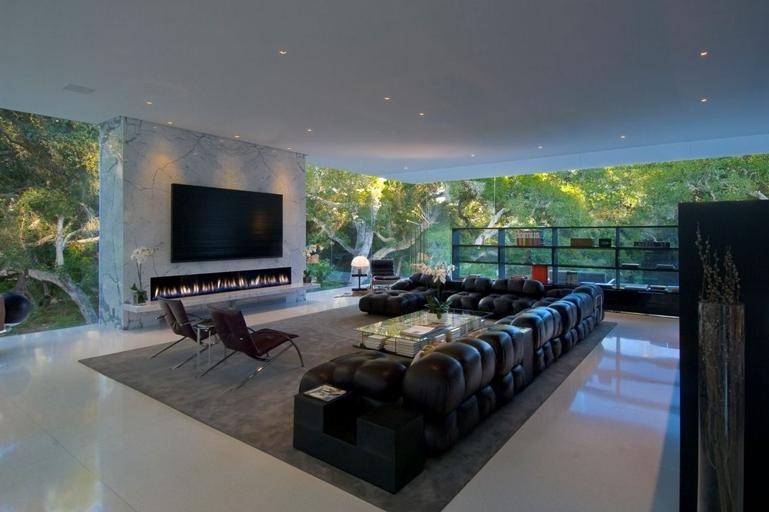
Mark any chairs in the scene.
[369,260,400,290]
[151,295,216,370]
[203,305,304,389]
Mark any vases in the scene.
[132,290,147,304]
[424,304,436,322]
[697,300,746,512]
[309,254,320,265]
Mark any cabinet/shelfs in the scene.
[450,221,680,318]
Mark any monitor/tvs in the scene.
[172,183,283,263]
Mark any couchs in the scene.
[293,272,604,496]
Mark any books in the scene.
[348,315,485,357]
[516,230,544,246]
[304,385,347,402]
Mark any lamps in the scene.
[350,256,370,291]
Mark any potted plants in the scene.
[428,297,450,324]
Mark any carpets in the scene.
[77,303,619,512]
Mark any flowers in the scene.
[129,247,153,291]
[304,243,323,258]
[694,226,748,512]
[411,260,457,302]
